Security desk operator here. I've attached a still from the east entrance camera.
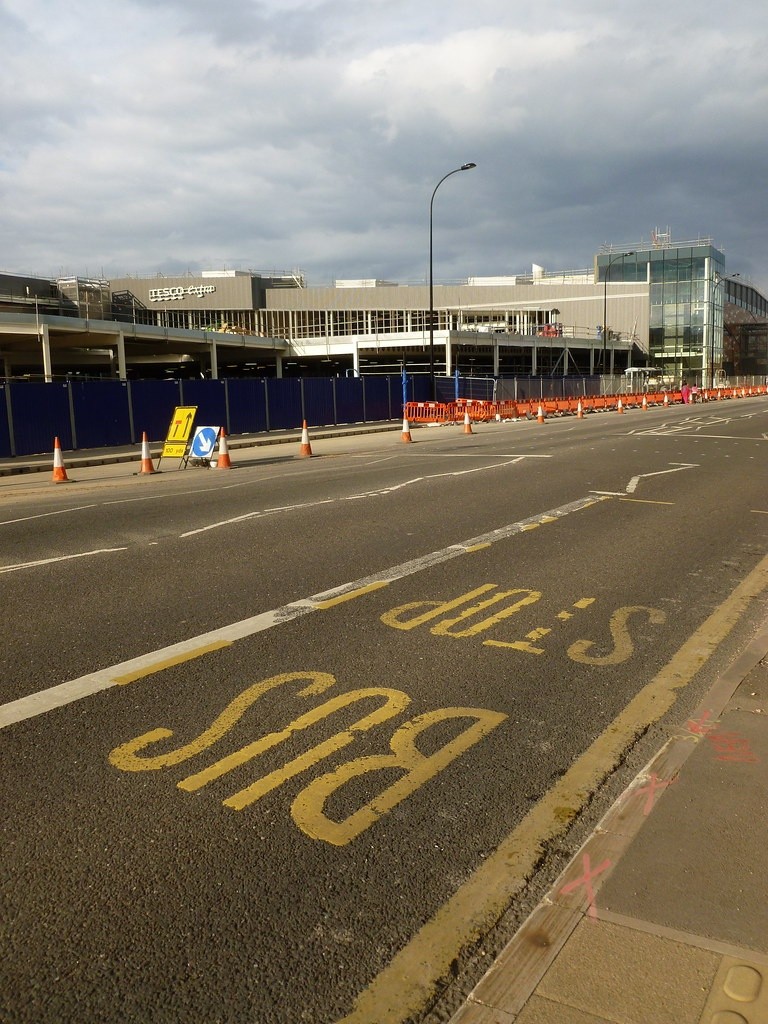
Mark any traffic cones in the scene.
[642,394,650,410]
[614,397,626,414]
[52,436,76,484]
[688,394,694,405]
[293,419,320,458]
[208,425,238,469]
[704,387,768,402]
[662,394,671,407]
[397,412,416,444]
[133,431,163,475]
[461,411,477,434]
[536,406,547,424]
[574,398,587,419]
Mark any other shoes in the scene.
[686,403,688,404]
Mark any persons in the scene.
[692,384,697,403]
[681,380,690,403]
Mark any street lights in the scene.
[429,162,476,401]
[603,252,634,375]
[712,273,740,387]
